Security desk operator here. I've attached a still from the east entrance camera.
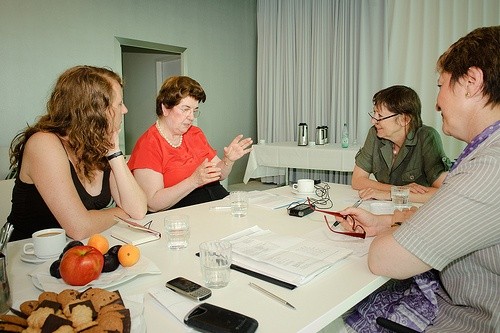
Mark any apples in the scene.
[59,245,104,286]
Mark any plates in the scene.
[30,275,134,294]
[20,238,70,264]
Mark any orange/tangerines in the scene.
[117,245,139,267]
[86,233,109,255]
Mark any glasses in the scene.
[306,197,366,240]
[368,110,402,124]
[177,104,200,118]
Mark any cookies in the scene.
[0,288,131,333]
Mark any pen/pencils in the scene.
[249,282,296,310]
[332,200,363,228]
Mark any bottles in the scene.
[260,137,265,145]
[342,123,349,148]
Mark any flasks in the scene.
[298,122,308,146]
[315,126,325,145]
[322,126,328,143]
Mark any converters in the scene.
[289,203,315,217]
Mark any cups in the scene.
[229,191,248,219]
[391,186,410,211]
[23,228,66,258]
[164,214,191,251]
[0,252,12,314]
[292,179,314,192]
[199,238,232,289]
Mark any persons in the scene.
[4,64,148,243]
[351,86,454,204]
[335,25,500,333]
[126,76,254,215]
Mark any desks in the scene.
[243,142,360,186]
[2,181,425,333]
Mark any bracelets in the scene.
[390,222,402,227]
[107,150,123,161]
[223,159,233,168]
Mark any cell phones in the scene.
[166,277,212,300]
[184,303,258,333]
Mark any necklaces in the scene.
[157,120,183,148]
[395,143,401,150]
